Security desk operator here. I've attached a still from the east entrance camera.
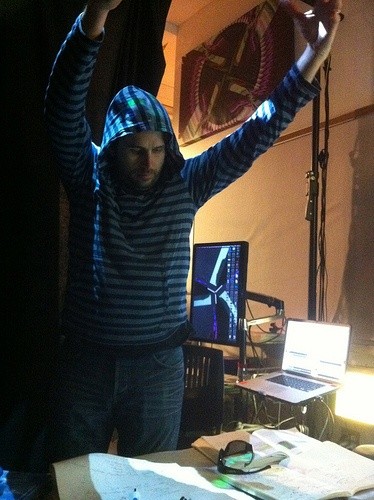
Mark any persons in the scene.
[44,0,345,463]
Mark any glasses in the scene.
[217,440,271,475]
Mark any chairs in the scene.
[178,344,224,448]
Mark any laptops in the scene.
[235,318,352,405]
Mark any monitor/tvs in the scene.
[188,241,248,345]
